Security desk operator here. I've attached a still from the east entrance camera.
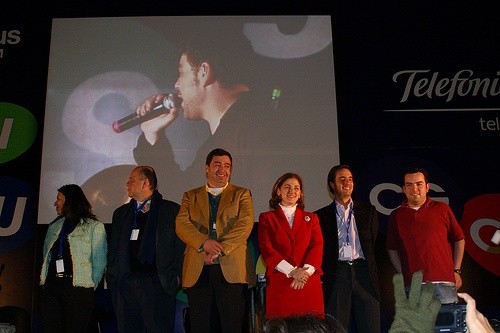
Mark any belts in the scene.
[55,273,73,278]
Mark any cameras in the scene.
[433,302,468,333]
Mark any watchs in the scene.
[454,268,462,274]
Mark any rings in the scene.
[302,277,304,280]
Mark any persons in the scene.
[313,165,382,333]
[175,149,254,333]
[264,313,346,333]
[258,173,324,319]
[386,168,496,333]
[40,184,108,333]
[133,36,299,222]
[106,165,187,333]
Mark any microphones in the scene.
[113,94,179,133]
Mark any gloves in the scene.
[387,270,442,333]
